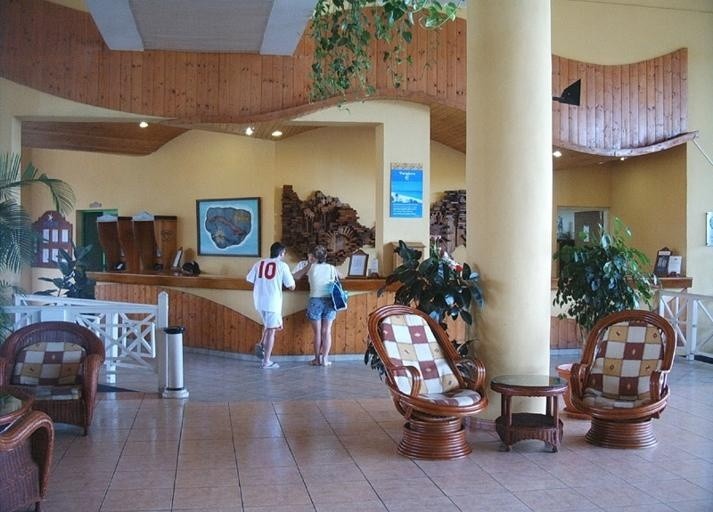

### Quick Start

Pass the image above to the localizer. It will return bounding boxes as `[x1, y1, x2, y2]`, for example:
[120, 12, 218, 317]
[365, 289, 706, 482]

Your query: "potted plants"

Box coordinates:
[551, 214, 658, 415]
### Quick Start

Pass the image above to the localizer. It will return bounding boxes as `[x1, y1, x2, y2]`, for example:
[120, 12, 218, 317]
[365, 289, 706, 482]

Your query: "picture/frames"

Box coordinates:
[195, 198, 262, 258]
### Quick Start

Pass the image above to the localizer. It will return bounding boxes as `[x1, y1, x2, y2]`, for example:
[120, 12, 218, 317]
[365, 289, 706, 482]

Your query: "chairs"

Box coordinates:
[0, 390, 55, 512]
[556, 309, 677, 449]
[368, 304, 489, 460]
[0, 320, 105, 435]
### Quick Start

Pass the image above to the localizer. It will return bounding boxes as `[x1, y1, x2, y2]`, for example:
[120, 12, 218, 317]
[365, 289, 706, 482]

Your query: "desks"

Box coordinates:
[491, 374, 567, 451]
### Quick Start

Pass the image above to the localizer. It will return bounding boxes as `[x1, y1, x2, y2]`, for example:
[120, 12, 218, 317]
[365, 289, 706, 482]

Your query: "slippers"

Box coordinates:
[255, 342, 264, 360]
[262, 361, 281, 370]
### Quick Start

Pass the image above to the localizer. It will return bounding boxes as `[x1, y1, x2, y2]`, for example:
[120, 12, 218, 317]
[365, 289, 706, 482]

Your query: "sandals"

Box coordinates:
[309, 360, 331, 368]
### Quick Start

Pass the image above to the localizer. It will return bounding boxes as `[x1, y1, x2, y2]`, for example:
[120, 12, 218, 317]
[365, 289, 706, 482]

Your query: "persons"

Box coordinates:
[435, 239, 462, 272]
[292, 250, 317, 281]
[305, 245, 338, 367]
[245, 242, 297, 369]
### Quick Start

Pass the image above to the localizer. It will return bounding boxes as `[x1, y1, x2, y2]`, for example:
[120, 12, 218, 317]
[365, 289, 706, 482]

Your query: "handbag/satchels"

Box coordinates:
[329, 279, 349, 313]
